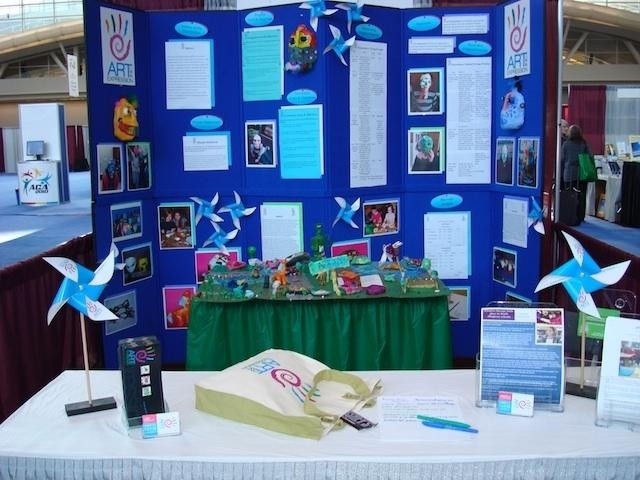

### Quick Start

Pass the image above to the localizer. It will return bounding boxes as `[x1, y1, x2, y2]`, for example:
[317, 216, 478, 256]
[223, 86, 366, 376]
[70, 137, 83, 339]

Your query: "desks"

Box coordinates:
[0, 370, 640, 480]
[190, 263, 451, 371]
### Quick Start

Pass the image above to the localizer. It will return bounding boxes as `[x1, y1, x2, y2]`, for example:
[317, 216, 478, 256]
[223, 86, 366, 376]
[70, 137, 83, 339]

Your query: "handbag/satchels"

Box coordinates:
[195, 348, 382, 440]
[578, 143, 598, 182]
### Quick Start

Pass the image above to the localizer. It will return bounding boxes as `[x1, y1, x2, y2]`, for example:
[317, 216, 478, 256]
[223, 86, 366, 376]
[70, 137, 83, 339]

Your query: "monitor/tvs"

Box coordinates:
[27, 141, 44, 160]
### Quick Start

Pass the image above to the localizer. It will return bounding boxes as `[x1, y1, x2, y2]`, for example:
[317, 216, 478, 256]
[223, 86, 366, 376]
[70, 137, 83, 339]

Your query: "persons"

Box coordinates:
[383, 205, 395, 226]
[536, 310, 561, 344]
[248, 134, 272, 163]
[559, 119, 570, 144]
[101, 153, 148, 236]
[161, 207, 177, 236]
[368, 208, 383, 225]
[411, 136, 438, 170]
[497, 144, 512, 184]
[178, 291, 190, 324]
[560, 125, 591, 221]
[172, 211, 188, 229]
[410, 73, 439, 112]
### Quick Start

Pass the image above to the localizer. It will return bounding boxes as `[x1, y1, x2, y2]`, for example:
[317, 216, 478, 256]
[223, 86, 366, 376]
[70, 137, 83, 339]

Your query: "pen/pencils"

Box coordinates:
[417, 415, 479, 433]
